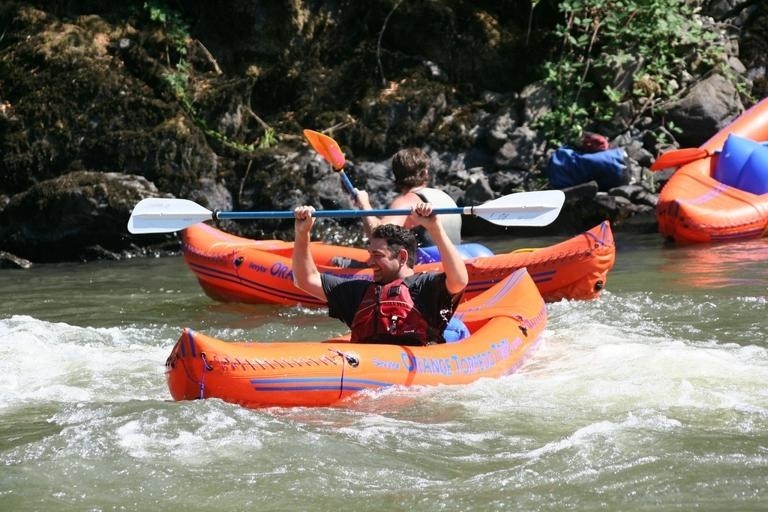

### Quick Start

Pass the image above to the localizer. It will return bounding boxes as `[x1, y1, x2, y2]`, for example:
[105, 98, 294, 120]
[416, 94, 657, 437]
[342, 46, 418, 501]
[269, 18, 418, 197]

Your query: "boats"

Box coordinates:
[655, 97, 768, 249]
[181, 221, 615, 310]
[165, 266, 547, 410]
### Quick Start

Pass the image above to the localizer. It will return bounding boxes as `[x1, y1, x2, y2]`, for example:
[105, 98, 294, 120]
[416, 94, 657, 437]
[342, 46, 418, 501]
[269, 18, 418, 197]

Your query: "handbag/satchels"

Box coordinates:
[547, 145, 633, 189]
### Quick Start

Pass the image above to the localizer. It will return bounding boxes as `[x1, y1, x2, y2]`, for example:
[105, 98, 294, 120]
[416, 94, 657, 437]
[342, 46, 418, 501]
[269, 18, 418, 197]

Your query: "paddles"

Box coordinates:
[126, 189, 565, 234]
[302, 128, 358, 202]
[650, 141, 768, 171]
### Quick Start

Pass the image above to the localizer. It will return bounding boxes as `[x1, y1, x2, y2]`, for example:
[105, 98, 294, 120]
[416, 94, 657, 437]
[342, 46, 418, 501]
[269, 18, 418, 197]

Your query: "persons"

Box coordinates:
[351, 148, 463, 245]
[292, 203, 470, 346]
